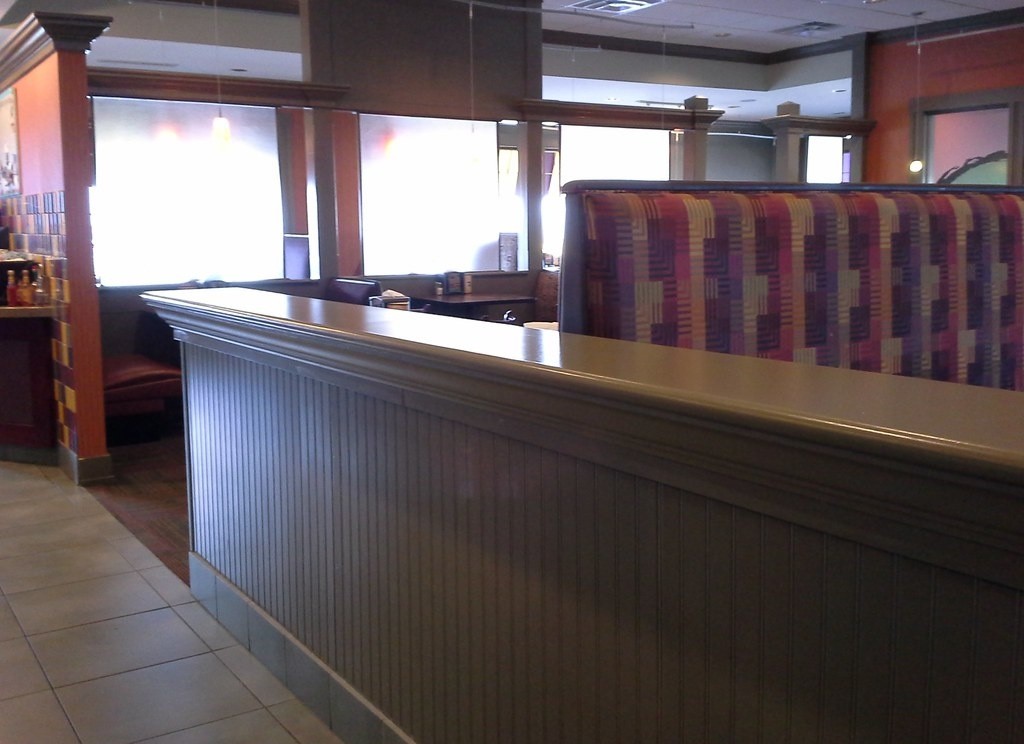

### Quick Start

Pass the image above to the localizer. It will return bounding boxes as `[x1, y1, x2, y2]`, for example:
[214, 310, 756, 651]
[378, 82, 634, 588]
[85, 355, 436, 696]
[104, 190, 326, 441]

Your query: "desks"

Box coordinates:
[409, 294, 538, 317]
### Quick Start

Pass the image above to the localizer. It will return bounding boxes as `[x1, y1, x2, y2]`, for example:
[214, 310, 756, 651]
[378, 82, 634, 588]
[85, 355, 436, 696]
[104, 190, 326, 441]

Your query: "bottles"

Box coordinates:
[436, 282, 444, 296]
[6, 264, 50, 306]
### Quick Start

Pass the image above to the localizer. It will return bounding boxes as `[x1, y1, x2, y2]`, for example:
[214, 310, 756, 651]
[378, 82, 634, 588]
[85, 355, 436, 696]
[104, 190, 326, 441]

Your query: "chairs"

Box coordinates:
[323, 275, 382, 305]
[532, 272, 560, 322]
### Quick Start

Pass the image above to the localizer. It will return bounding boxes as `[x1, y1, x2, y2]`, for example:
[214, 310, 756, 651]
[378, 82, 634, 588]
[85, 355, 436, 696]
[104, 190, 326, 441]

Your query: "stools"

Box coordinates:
[104, 346, 182, 416]
